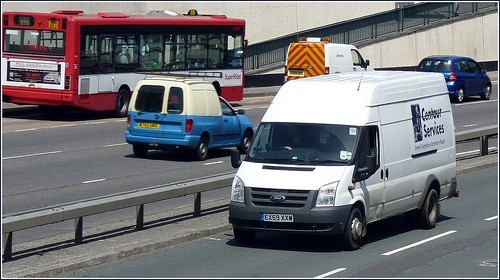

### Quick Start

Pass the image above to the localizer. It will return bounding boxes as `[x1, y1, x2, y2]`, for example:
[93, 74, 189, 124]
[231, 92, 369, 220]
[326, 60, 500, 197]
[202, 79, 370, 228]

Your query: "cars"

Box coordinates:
[415, 55, 492, 103]
[126, 75, 256, 160]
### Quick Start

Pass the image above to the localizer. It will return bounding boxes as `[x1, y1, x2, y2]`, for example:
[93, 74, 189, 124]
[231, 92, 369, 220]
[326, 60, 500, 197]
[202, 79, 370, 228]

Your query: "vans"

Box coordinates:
[228, 70, 459, 250]
[284, 36, 374, 83]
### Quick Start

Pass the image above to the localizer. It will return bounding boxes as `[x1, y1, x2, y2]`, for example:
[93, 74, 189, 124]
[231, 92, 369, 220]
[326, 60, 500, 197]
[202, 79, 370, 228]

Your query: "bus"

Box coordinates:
[1, 9, 249, 117]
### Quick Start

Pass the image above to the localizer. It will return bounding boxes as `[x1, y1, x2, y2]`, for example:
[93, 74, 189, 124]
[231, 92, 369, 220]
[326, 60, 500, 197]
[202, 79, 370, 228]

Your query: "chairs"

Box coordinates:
[9, 38, 224, 74]
